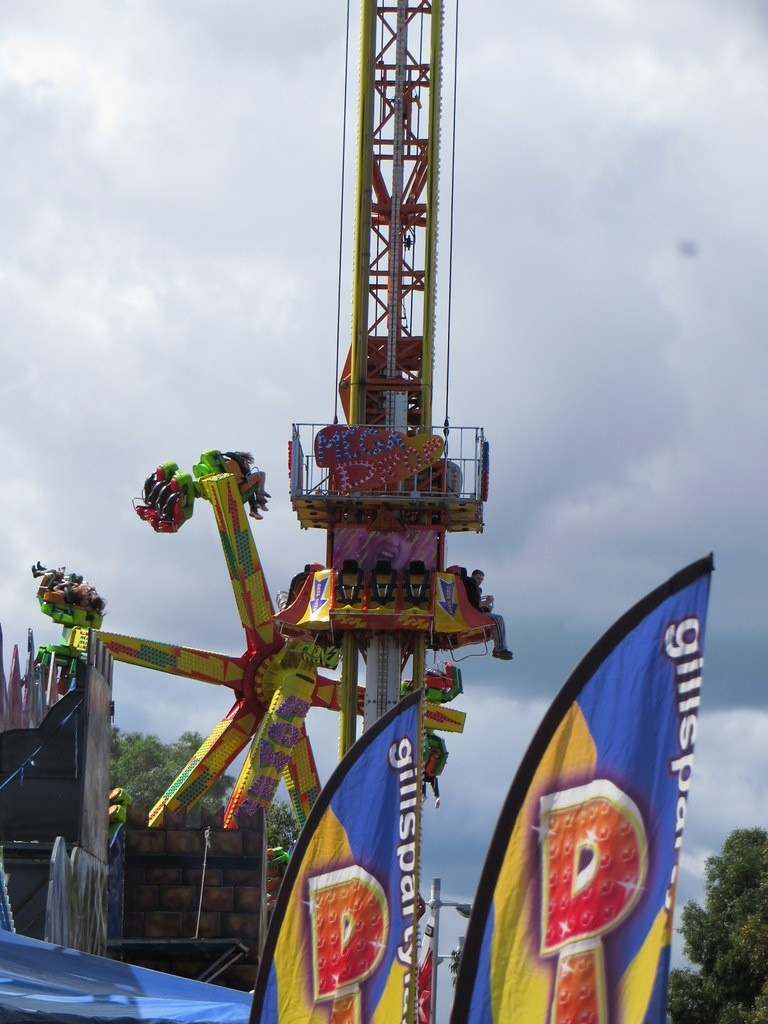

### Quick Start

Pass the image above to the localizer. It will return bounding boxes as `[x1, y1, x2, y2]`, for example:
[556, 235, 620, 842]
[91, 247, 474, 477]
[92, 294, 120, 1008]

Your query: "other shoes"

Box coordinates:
[31, 565, 42, 579]
[254, 490, 272, 503]
[36, 561, 45, 571]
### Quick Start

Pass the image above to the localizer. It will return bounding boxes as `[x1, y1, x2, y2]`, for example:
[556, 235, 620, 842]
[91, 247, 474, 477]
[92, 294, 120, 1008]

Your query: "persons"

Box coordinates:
[468, 570, 513, 660]
[32, 561, 105, 611]
[235, 452, 271, 519]
[423, 727, 447, 805]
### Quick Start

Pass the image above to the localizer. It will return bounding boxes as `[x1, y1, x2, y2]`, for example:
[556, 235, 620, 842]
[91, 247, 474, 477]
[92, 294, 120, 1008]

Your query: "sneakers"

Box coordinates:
[254, 499, 270, 512]
[248, 510, 264, 520]
[434, 797, 441, 809]
[492, 649, 514, 660]
